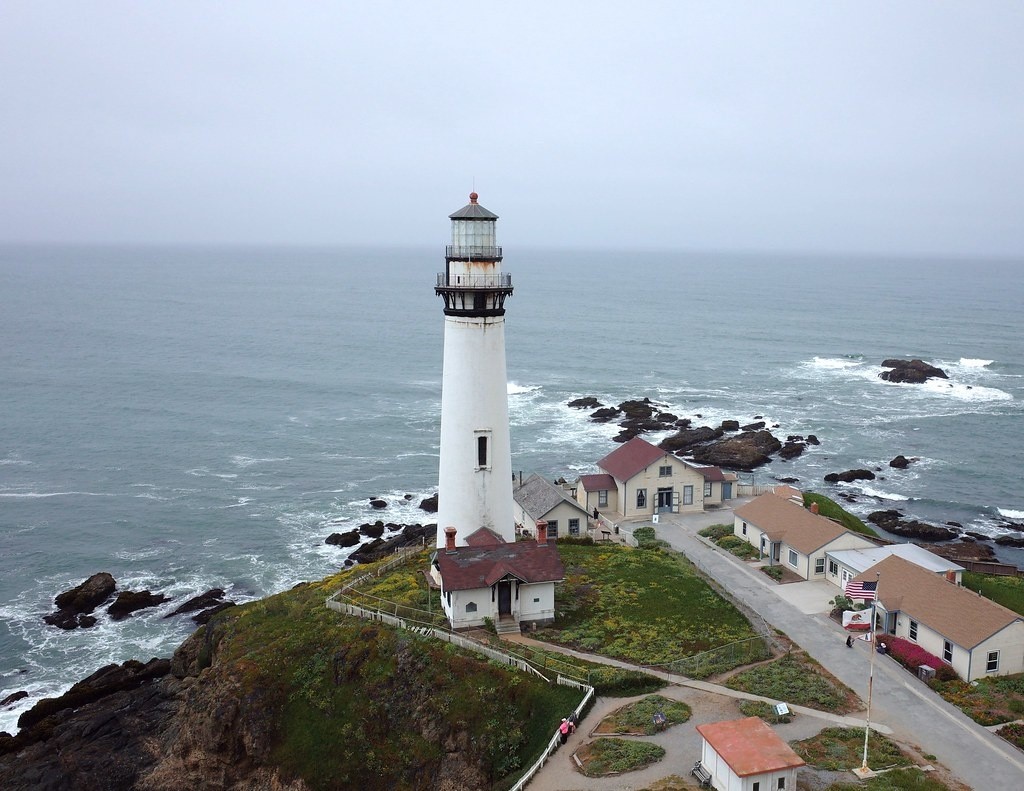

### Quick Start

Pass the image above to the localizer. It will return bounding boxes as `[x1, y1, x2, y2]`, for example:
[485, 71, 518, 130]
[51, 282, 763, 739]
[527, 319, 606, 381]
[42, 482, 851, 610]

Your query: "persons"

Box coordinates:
[846, 636, 855, 648]
[560, 718, 568, 744]
[593, 507, 599, 524]
[569, 710, 577, 734]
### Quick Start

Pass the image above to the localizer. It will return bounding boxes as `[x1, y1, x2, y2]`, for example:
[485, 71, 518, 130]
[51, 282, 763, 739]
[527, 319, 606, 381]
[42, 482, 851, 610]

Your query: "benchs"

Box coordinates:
[691, 763, 712, 789]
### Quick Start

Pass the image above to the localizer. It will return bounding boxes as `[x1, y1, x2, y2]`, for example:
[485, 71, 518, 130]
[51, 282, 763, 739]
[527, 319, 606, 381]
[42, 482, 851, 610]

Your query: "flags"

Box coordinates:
[845, 581, 878, 600]
[842, 608, 872, 629]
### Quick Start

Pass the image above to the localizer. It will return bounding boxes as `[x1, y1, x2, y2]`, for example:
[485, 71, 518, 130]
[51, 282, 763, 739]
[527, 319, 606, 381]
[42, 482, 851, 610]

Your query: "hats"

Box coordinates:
[562, 718, 566, 721]
